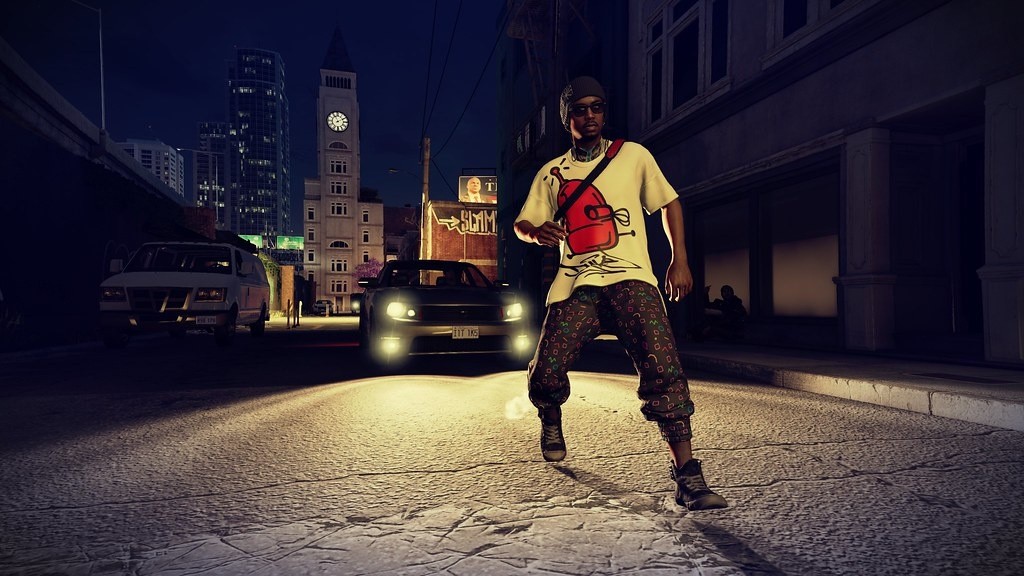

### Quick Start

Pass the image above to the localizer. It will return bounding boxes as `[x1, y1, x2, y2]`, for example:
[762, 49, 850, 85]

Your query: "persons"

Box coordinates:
[460, 176, 490, 202]
[439, 264, 467, 287]
[714, 284, 747, 317]
[512, 76, 729, 511]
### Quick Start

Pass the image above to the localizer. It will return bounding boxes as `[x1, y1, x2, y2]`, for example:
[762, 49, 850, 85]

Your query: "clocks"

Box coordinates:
[326, 111, 349, 133]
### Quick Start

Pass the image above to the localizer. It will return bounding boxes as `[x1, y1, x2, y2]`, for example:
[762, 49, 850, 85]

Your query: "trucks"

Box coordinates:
[350, 293, 364, 314]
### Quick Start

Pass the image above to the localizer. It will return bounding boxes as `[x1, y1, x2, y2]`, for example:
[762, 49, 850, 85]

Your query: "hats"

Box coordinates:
[560, 76, 606, 133]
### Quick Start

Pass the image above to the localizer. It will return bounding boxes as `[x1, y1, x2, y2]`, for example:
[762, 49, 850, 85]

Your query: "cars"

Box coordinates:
[312, 300, 334, 315]
[356, 260, 532, 369]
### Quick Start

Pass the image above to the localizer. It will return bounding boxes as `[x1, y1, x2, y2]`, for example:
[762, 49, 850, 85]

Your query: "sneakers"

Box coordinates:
[538, 405, 567, 462]
[668, 458, 728, 510]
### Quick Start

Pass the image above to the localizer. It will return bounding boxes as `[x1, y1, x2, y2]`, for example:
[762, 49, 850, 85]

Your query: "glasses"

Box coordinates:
[569, 101, 606, 116]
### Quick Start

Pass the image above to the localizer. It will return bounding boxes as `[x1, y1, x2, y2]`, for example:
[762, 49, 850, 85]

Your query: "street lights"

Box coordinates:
[389, 168, 424, 259]
[177, 148, 224, 221]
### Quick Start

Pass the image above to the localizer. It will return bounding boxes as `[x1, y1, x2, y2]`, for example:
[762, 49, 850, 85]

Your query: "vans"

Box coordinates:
[98, 242, 271, 347]
